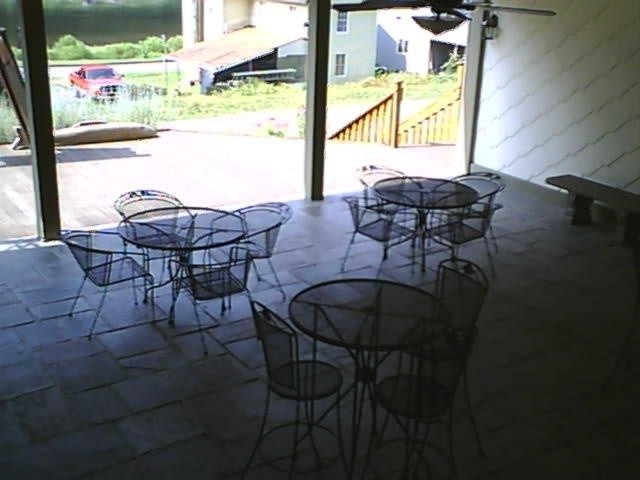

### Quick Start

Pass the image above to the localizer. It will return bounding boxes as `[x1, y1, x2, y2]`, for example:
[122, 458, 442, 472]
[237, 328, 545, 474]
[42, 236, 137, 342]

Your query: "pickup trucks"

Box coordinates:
[70, 63, 127, 103]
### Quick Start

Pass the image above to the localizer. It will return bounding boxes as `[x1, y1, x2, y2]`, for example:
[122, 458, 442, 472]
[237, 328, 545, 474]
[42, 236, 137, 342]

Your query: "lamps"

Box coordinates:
[480, 13, 499, 41]
[409, 14, 467, 34]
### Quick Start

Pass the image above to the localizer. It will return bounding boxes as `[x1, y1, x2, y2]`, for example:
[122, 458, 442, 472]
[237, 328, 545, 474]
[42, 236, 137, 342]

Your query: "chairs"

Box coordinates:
[209, 201, 294, 310]
[420, 201, 504, 284]
[428, 170, 506, 258]
[358, 164, 425, 247]
[61, 227, 157, 340]
[240, 299, 349, 479]
[359, 323, 481, 478]
[341, 195, 417, 282]
[389, 257, 491, 453]
[166, 240, 272, 357]
[113, 189, 197, 303]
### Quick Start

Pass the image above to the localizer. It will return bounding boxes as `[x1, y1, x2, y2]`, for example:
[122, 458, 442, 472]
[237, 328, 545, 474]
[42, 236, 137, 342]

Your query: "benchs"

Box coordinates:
[545, 173, 640, 244]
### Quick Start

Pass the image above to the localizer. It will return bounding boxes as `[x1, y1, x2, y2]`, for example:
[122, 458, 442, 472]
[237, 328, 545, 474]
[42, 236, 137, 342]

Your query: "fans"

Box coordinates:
[331, 0, 556, 22]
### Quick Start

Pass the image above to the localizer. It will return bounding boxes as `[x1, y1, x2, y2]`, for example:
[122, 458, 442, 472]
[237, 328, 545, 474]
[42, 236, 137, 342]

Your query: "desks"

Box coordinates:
[116, 205, 248, 326]
[370, 177, 481, 273]
[287, 277, 452, 478]
